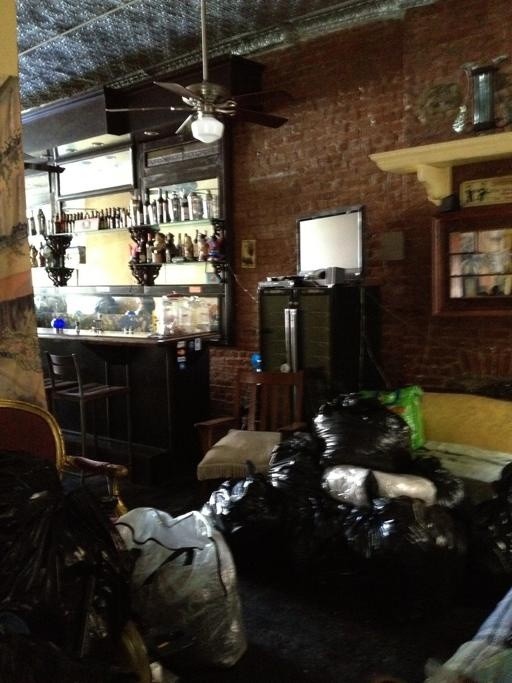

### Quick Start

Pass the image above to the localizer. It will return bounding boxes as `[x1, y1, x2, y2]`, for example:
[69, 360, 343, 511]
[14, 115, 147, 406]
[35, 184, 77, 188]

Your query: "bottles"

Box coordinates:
[25, 184, 220, 266]
[155, 294, 213, 337]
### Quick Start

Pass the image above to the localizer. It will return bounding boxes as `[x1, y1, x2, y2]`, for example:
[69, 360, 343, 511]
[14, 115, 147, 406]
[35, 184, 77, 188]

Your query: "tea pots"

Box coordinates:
[464, 54, 505, 132]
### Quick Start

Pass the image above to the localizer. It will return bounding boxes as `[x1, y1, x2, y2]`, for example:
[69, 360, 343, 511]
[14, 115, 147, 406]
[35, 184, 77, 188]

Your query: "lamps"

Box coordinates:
[188, 105, 226, 143]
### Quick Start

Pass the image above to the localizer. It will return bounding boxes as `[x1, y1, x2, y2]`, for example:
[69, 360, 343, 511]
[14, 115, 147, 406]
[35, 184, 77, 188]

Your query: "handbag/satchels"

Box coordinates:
[114, 506, 248, 669]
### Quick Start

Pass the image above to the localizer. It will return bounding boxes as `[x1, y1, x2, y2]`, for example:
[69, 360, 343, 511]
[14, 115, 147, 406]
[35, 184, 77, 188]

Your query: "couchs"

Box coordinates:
[412, 386, 512, 491]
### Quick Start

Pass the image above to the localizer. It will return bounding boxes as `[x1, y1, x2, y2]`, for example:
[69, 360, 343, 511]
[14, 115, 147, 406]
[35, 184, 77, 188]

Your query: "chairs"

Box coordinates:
[192, 367, 306, 483]
[0, 396, 132, 526]
[45, 349, 135, 471]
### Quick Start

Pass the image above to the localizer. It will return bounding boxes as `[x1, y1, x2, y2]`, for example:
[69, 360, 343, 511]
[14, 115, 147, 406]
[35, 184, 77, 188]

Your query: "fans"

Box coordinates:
[104, 0, 294, 137]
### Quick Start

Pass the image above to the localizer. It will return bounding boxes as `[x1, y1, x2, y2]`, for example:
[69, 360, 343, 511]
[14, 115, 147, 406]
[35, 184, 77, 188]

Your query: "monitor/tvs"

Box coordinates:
[296, 204, 365, 277]
